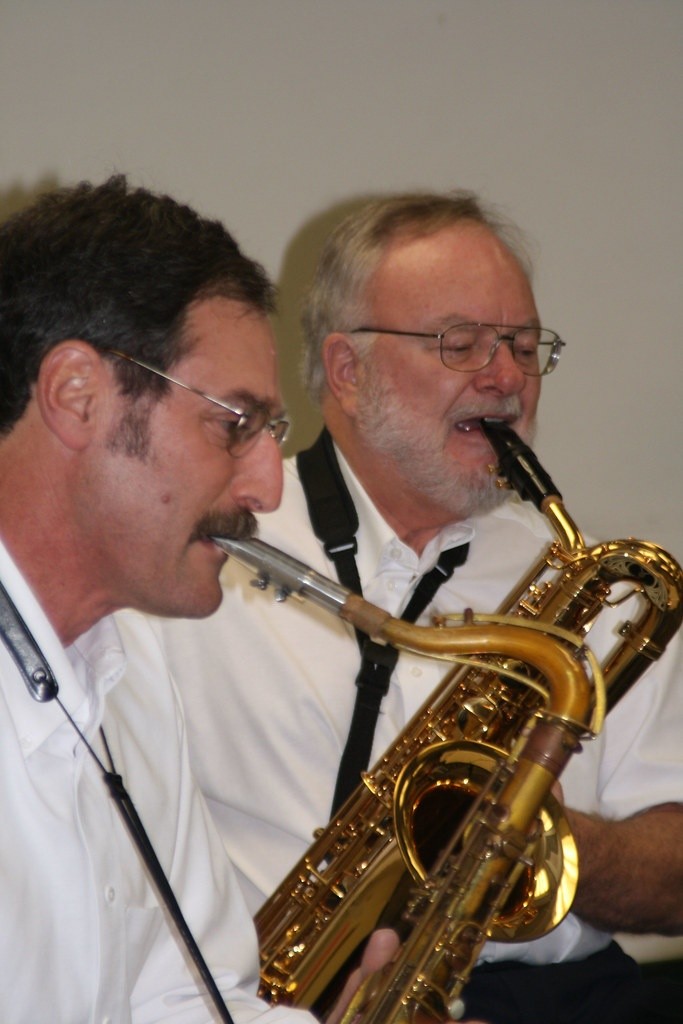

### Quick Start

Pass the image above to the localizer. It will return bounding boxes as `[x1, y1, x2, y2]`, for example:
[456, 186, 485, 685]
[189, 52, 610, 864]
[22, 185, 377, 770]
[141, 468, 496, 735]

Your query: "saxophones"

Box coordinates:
[252, 419, 683, 1023]
[211, 538, 605, 1024]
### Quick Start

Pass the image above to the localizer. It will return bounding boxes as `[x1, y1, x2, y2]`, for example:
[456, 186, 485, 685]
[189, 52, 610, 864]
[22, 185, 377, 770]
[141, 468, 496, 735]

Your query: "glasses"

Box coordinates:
[349, 320, 566, 379]
[101, 347, 290, 460]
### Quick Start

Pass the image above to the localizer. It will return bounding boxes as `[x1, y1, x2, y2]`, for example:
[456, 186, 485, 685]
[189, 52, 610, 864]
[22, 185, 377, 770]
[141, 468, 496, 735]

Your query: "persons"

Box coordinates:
[158, 189, 683, 1024]
[1, 173, 446, 1024]
